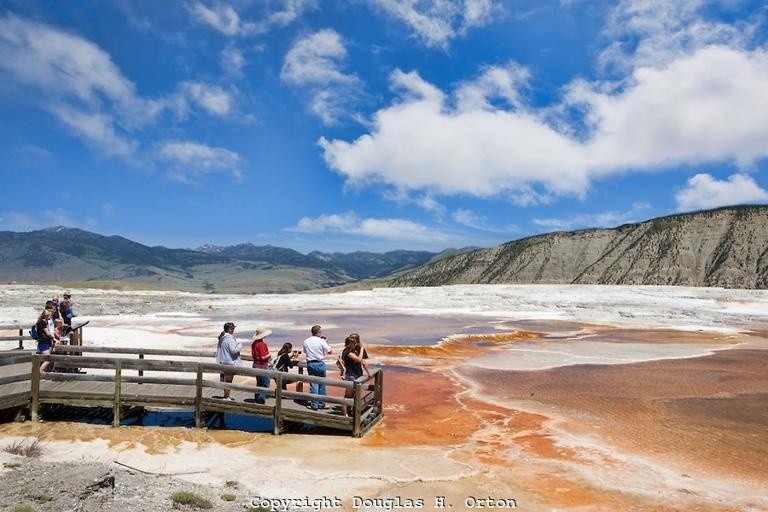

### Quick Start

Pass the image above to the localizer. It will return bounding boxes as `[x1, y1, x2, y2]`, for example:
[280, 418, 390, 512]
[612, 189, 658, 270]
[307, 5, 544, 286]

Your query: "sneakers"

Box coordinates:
[319, 404, 329, 409]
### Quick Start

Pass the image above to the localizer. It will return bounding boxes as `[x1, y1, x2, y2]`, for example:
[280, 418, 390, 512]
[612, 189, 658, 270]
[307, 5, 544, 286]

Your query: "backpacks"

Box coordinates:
[29, 325, 39, 340]
[269, 353, 284, 370]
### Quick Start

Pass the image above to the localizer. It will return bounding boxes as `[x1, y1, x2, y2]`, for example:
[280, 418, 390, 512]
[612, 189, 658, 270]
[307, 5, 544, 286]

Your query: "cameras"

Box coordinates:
[320, 336, 326, 340]
[294, 351, 301, 354]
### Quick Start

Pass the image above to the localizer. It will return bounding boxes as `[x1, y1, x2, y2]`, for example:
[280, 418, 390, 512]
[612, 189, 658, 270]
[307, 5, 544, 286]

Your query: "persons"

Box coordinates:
[36, 291, 81, 373]
[273, 343, 298, 389]
[348, 333, 372, 378]
[302, 325, 332, 411]
[341, 337, 373, 417]
[251, 327, 273, 404]
[215, 322, 242, 401]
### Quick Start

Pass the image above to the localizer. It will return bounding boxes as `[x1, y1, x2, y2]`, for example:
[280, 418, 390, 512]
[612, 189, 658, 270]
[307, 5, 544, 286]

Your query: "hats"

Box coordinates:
[223, 323, 237, 329]
[252, 327, 272, 340]
